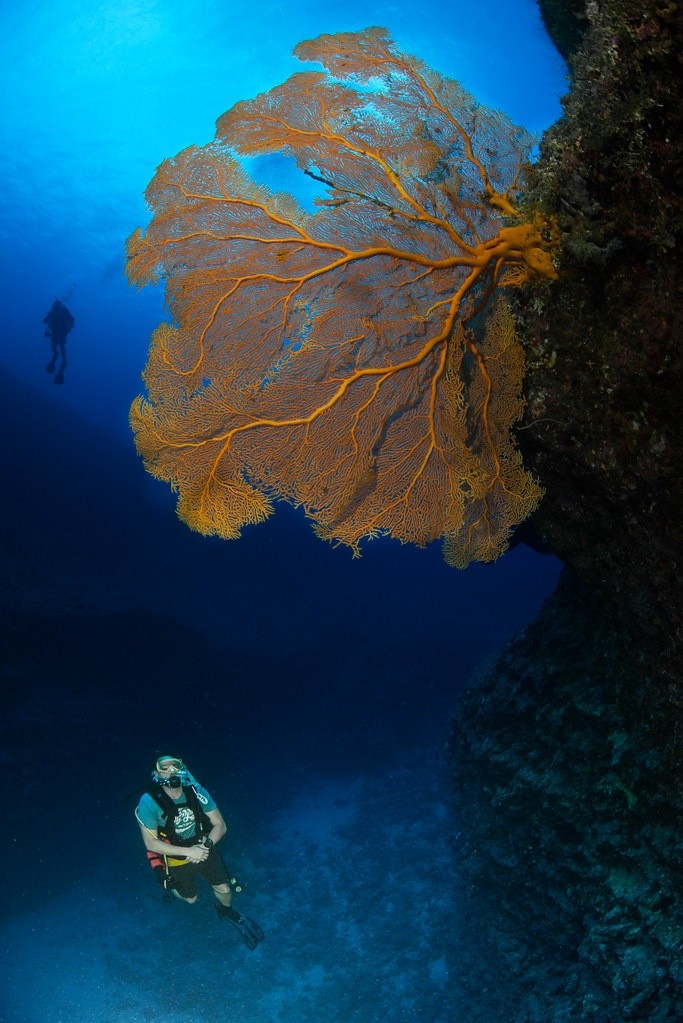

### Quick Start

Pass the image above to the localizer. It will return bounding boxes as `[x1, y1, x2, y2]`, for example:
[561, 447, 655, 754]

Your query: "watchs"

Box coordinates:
[203, 838, 214, 849]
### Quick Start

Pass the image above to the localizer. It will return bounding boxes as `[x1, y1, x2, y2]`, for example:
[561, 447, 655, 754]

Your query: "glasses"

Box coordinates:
[156, 759, 183, 773]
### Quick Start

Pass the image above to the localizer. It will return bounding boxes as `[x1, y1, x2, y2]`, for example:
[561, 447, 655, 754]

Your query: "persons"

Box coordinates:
[135, 756, 265, 951]
[44, 300, 75, 385]
